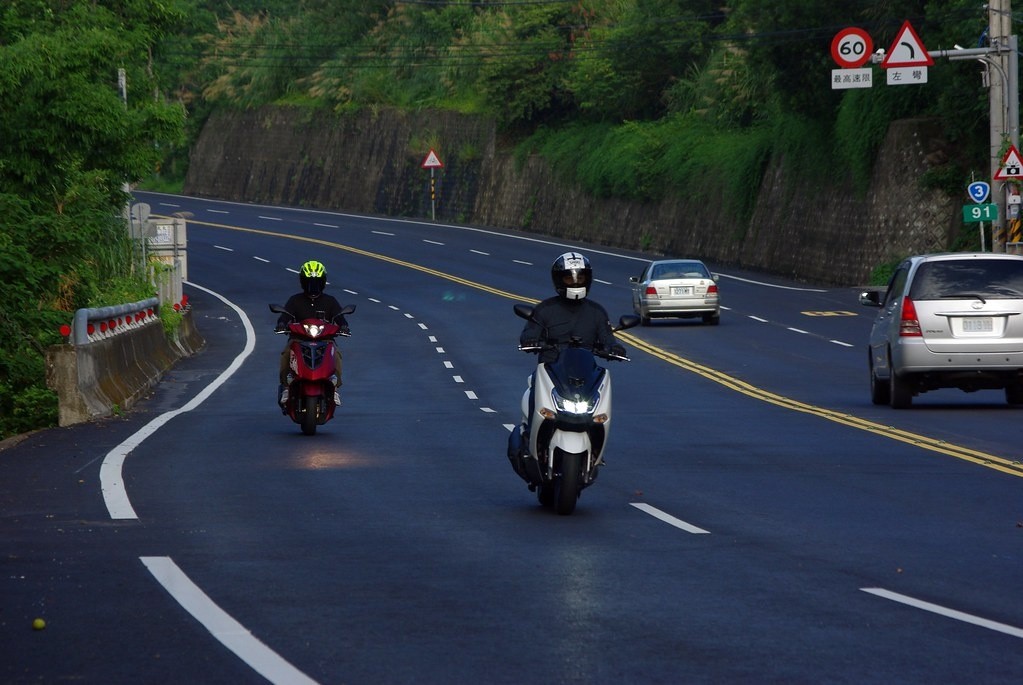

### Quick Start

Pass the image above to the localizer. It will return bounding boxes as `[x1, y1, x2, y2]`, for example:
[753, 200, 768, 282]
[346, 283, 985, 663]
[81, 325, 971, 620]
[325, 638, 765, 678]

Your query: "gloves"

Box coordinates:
[608, 344, 627, 362]
[340, 326, 351, 338]
[275, 323, 289, 336]
[520, 339, 539, 356]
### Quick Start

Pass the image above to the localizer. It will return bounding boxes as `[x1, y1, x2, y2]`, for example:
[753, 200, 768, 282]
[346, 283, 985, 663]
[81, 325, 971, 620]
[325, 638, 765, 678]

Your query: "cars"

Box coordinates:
[628, 259, 722, 325]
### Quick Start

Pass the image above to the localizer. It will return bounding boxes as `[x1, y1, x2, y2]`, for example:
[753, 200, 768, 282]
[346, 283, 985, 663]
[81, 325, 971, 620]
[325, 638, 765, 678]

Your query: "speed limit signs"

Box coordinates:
[831, 27, 874, 69]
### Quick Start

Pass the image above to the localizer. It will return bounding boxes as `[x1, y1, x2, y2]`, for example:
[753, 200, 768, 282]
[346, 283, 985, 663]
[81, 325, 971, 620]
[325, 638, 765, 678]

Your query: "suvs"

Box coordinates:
[857, 252, 1023, 408]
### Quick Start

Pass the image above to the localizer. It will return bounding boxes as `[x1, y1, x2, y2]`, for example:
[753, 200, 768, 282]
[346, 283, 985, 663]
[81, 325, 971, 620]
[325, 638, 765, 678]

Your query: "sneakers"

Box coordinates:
[280, 389, 290, 403]
[334, 391, 341, 406]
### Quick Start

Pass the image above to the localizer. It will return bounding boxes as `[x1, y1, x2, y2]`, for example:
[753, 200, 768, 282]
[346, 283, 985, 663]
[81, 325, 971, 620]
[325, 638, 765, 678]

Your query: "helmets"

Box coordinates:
[550, 252, 593, 301]
[299, 260, 327, 294]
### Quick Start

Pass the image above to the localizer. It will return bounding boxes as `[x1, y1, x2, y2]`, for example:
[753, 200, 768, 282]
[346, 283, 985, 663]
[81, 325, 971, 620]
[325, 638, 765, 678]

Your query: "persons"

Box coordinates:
[276, 261, 351, 405]
[520, 252, 627, 467]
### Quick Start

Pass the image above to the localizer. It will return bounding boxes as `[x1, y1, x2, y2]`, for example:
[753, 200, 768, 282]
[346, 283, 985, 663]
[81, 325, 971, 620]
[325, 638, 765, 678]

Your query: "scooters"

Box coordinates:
[268, 303, 357, 436]
[506, 302, 642, 517]
